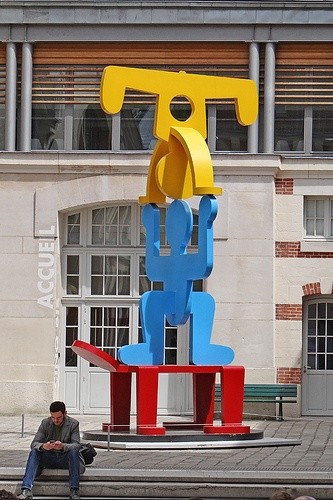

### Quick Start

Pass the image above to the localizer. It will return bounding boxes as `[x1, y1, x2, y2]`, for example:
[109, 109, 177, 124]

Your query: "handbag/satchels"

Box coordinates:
[77, 442, 98, 474]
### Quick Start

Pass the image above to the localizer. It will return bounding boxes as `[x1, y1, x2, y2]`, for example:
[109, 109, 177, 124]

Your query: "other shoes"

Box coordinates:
[70, 488, 80, 500]
[16, 488, 33, 500]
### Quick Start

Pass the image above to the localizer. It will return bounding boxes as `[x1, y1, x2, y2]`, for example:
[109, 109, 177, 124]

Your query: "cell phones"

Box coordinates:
[50, 440, 55, 444]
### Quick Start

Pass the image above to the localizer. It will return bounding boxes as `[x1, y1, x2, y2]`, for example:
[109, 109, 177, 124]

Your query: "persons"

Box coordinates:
[18, 401, 88, 500]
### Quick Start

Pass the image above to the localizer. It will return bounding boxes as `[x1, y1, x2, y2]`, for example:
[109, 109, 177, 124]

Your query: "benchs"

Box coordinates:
[214, 384, 297, 420]
